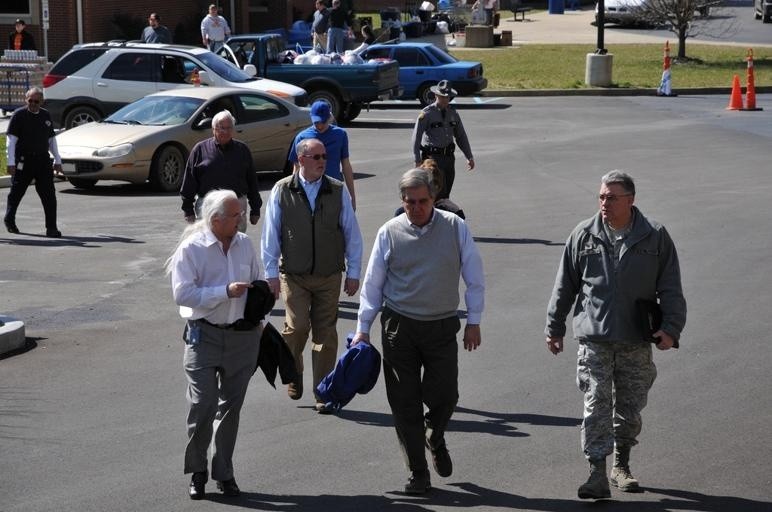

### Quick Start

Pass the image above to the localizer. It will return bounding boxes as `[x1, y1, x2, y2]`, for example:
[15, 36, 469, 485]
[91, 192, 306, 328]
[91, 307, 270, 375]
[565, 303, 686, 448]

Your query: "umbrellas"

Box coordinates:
[218, 479, 240, 496]
[189, 479, 204, 499]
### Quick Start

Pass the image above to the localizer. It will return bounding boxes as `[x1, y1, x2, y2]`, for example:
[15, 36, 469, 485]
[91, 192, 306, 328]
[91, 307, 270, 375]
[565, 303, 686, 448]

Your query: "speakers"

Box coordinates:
[578, 460, 610, 498]
[610, 454, 638, 491]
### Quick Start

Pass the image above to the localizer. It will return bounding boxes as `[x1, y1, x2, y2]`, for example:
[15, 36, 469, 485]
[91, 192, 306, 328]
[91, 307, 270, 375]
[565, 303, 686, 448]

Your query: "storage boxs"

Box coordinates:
[510, 7, 533, 21]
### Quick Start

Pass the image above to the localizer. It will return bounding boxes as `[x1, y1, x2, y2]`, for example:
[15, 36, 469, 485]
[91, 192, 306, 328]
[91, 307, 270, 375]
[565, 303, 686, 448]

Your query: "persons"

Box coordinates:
[4, 87, 61, 239]
[543, 168, 687, 500]
[410, 80, 475, 202]
[260, 140, 364, 414]
[393, 159, 465, 221]
[311, 1, 497, 56]
[201, 3, 231, 53]
[350, 167, 485, 494]
[140, 12, 172, 64]
[8, 19, 35, 49]
[287, 97, 356, 213]
[179, 109, 263, 234]
[164, 188, 266, 497]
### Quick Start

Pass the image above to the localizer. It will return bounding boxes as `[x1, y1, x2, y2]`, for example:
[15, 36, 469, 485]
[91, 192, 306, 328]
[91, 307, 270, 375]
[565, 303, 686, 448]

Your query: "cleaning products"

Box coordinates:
[3, 69, 37, 112]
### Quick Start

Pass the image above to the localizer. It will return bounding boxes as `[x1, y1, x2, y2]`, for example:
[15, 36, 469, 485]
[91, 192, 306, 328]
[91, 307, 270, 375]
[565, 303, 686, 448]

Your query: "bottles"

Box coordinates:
[311, 101, 328, 122]
[431, 80, 457, 97]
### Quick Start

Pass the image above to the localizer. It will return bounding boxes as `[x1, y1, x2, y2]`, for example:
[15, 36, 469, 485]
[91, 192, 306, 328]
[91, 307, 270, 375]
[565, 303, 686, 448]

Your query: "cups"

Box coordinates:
[303, 154, 328, 160]
[600, 194, 628, 200]
[27, 98, 39, 104]
[224, 210, 244, 217]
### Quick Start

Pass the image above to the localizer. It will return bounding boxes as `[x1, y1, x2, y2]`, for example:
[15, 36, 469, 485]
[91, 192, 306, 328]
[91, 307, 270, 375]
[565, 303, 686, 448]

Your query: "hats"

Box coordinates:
[593, 0, 709, 24]
[353, 37, 487, 107]
[38, 84, 323, 189]
[747, 3, 772, 24]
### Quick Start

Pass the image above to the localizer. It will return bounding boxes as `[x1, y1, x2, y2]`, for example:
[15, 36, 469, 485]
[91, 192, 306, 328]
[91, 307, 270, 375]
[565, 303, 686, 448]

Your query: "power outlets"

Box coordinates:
[211, 32, 398, 119]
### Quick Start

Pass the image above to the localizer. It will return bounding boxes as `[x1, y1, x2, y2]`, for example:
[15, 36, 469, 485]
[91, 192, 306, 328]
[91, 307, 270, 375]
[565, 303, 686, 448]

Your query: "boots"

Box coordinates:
[45, 39, 301, 128]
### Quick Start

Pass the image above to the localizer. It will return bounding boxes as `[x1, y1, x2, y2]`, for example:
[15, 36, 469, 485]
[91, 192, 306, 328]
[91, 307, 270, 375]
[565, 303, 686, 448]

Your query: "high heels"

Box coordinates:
[724, 76, 743, 112]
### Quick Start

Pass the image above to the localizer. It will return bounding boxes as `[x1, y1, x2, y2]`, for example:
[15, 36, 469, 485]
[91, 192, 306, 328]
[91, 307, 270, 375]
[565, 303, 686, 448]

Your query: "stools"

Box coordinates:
[432, 147, 450, 154]
[199, 318, 237, 329]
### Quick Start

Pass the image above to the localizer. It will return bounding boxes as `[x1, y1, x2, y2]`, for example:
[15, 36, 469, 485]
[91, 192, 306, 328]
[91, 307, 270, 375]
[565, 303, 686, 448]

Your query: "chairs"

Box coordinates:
[426, 437, 452, 476]
[46, 229, 61, 237]
[4, 218, 19, 232]
[405, 471, 430, 492]
[288, 374, 302, 399]
[316, 401, 325, 412]
